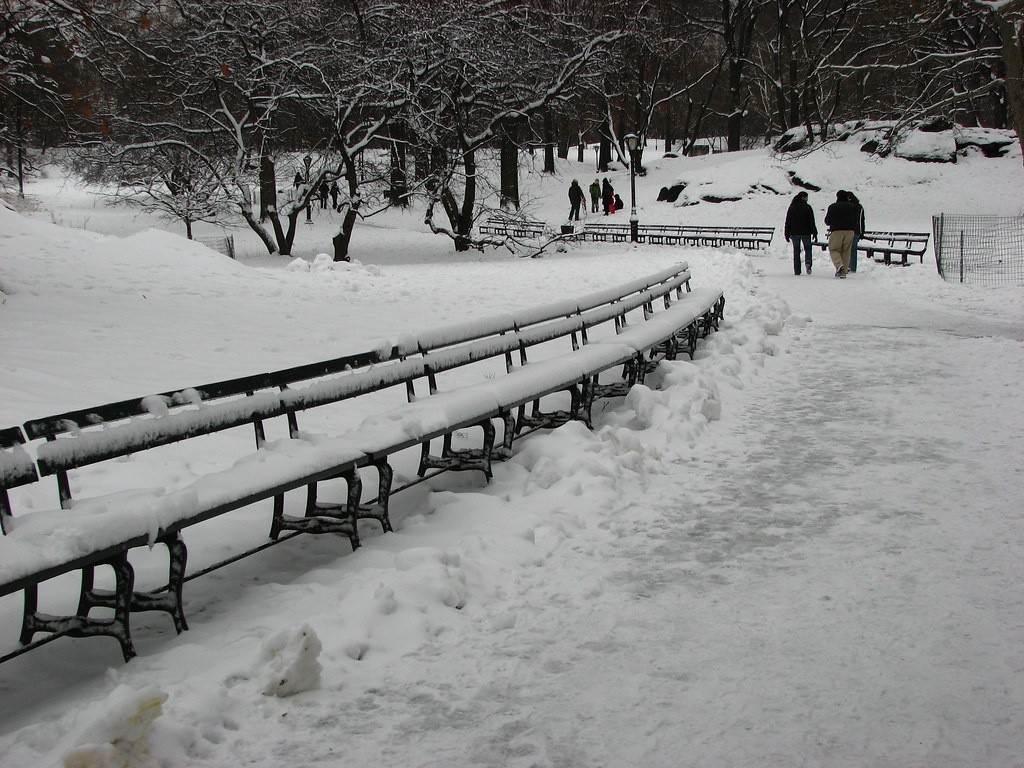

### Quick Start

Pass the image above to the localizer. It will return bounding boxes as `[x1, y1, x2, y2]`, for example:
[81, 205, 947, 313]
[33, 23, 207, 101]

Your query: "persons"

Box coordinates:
[330, 181, 342, 209]
[784, 191, 818, 275]
[846, 191, 865, 273]
[293, 172, 304, 190]
[589, 179, 601, 213]
[318, 181, 329, 209]
[600, 178, 615, 216]
[568, 179, 586, 221]
[602, 194, 623, 213]
[825, 190, 857, 278]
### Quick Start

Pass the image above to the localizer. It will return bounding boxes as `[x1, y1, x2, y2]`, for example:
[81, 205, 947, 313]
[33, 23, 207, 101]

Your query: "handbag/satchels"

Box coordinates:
[609, 202, 616, 213]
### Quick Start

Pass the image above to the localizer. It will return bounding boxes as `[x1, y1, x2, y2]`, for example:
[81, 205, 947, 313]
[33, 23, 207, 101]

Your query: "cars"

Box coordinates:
[918, 116, 954, 132]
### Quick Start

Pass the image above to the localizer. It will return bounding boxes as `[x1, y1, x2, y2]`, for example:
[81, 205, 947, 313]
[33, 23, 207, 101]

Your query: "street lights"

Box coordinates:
[304, 155, 314, 225]
[624, 134, 642, 245]
[593, 144, 599, 169]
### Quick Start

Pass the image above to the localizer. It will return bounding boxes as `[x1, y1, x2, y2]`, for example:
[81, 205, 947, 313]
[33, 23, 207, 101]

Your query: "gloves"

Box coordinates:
[812, 234, 818, 243]
[786, 235, 790, 242]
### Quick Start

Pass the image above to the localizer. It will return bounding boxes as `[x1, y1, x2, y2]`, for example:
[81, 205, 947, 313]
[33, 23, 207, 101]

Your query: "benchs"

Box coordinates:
[0, 217, 931, 665]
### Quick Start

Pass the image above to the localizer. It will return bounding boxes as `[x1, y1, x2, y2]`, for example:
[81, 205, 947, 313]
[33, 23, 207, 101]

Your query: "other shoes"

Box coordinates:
[833, 266, 845, 277]
[847, 267, 855, 273]
[805, 263, 811, 275]
[840, 277, 846, 278]
